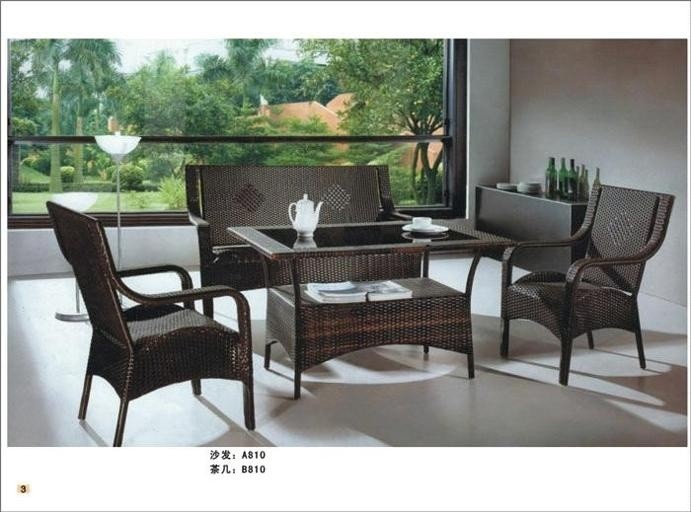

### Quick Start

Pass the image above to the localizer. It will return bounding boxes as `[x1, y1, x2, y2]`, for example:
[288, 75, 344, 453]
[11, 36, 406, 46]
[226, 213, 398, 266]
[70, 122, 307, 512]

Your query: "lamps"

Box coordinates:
[50, 189, 99, 321]
[93, 129, 143, 271]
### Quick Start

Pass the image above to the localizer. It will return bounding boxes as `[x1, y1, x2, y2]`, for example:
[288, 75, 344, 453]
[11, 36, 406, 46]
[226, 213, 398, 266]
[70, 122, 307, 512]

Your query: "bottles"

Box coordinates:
[544, 156, 578, 202]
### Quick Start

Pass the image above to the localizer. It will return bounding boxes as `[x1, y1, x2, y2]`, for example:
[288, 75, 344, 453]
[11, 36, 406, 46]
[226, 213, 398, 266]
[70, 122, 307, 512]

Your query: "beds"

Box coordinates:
[224, 218, 518, 400]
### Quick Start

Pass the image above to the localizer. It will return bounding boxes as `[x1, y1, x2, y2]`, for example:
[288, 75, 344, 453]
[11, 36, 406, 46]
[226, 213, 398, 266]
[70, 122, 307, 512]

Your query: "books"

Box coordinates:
[306, 279, 418, 303]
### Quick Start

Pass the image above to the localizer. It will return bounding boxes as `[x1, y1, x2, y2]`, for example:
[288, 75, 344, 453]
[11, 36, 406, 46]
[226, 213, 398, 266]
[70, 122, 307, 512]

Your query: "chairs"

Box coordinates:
[45, 199, 255, 447]
[500, 183, 676, 387]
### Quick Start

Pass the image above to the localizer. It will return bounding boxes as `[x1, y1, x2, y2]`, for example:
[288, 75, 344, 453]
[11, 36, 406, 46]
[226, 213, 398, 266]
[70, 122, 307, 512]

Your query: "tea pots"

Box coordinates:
[288, 192, 323, 237]
[292, 237, 316, 249]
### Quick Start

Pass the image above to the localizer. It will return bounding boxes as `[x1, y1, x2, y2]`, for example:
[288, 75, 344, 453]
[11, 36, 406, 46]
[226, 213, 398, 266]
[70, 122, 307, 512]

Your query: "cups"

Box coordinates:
[411, 217, 432, 229]
[411, 239, 431, 243]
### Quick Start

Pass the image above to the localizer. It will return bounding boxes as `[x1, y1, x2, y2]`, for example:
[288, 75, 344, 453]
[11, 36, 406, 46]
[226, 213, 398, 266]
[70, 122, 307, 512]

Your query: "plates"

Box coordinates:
[400, 232, 450, 240]
[495, 181, 540, 195]
[401, 223, 450, 234]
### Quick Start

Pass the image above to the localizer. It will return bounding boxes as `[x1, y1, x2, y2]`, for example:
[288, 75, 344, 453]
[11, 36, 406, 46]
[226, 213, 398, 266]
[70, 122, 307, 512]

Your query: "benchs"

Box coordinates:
[184, 164, 419, 330]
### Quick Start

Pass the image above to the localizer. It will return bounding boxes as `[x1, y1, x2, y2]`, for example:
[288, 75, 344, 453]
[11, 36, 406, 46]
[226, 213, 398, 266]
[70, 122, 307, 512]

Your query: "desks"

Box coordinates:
[474, 184, 589, 274]
